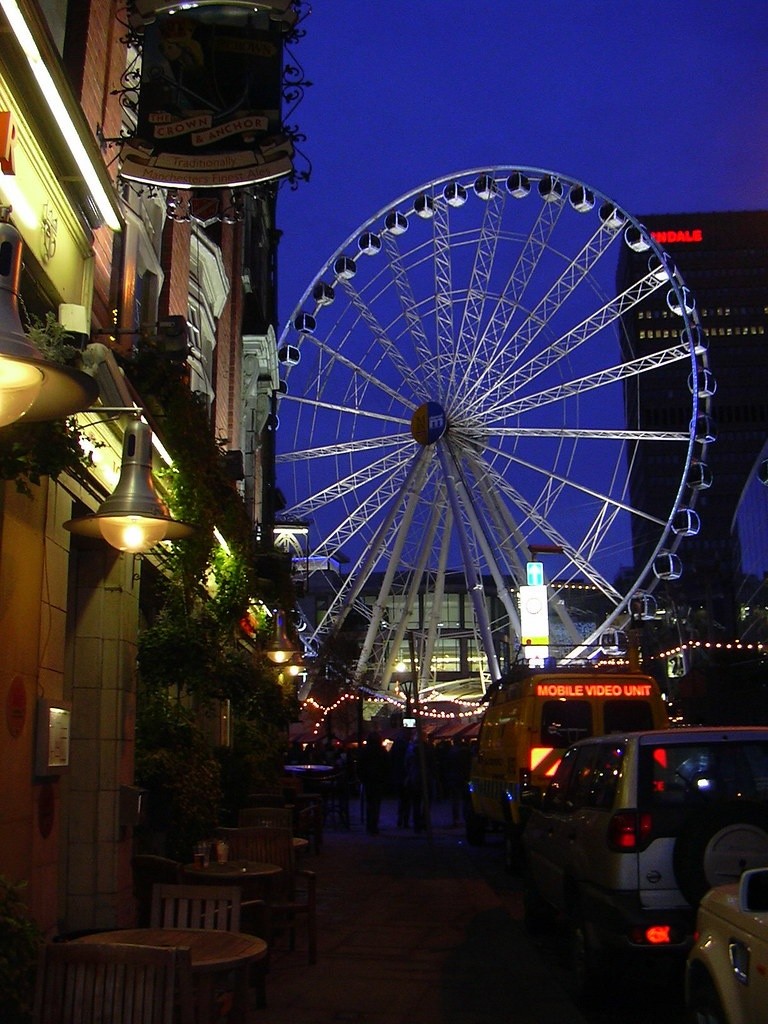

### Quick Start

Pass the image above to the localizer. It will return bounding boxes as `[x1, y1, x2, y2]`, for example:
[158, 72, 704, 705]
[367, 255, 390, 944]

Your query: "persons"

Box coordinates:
[305, 732, 476, 833]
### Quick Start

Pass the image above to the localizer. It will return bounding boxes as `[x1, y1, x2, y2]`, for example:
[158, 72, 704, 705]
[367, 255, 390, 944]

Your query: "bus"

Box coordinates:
[464, 655, 671, 878]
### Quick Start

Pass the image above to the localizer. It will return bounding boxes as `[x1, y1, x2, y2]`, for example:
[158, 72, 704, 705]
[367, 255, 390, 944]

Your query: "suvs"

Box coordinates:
[514, 722, 767, 986]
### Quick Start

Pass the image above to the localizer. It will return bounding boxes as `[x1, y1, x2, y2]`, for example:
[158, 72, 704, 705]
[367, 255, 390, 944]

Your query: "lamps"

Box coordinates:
[0, 216, 102, 431]
[274, 641, 312, 677]
[63, 396, 205, 551]
[234, 601, 297, 663]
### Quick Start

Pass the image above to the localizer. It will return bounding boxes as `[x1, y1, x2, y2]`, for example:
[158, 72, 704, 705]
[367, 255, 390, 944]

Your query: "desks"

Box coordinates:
[214, 827, 290, 852]
[179, 859, 280, 935]
[235, 835, 309, 866]
[67, 928, 269, 1024]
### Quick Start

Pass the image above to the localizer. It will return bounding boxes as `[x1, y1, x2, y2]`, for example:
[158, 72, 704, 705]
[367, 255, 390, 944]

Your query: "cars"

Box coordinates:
[682, 867, 768, 1024]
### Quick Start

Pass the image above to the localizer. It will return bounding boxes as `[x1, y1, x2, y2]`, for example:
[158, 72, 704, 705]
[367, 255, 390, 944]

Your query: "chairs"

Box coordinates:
[29, 779, 330, 1023]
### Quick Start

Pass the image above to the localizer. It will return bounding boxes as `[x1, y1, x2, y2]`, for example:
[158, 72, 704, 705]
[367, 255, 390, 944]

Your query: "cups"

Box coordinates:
[215, 840, 229, 865]
[198, 841, 212, 868]
[194, 854, 205, 868]
